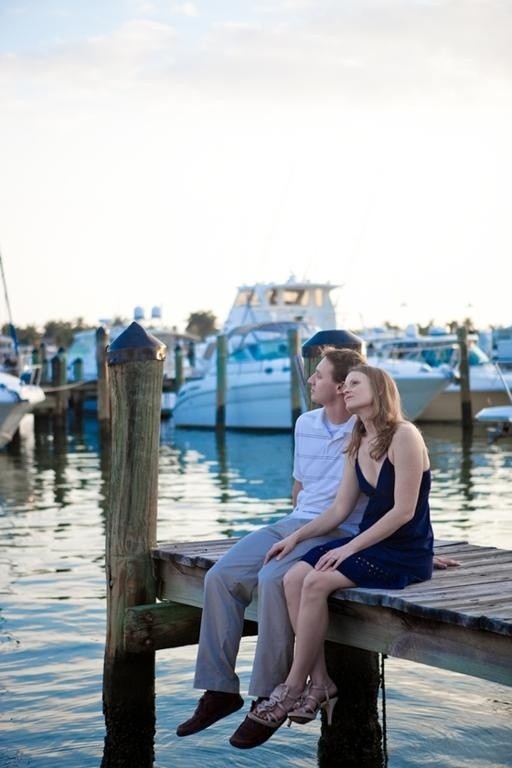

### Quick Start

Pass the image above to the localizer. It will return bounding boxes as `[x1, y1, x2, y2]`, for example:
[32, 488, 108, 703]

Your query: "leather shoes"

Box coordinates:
[228, 695, 288, 749]
[176, 690, 244, 737]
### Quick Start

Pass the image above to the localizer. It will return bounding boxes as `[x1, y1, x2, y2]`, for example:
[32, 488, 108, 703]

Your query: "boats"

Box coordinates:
[0, 363, 49, 455]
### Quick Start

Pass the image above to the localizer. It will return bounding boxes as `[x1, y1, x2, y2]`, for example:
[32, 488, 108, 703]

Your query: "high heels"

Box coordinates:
[247, 681, 310, 729]
[286, 676, 340, 727]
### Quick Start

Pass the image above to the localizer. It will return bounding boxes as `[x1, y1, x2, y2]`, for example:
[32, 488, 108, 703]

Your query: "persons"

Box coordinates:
[247, 364, 434, 728]
[176, 345, 368, 749]
